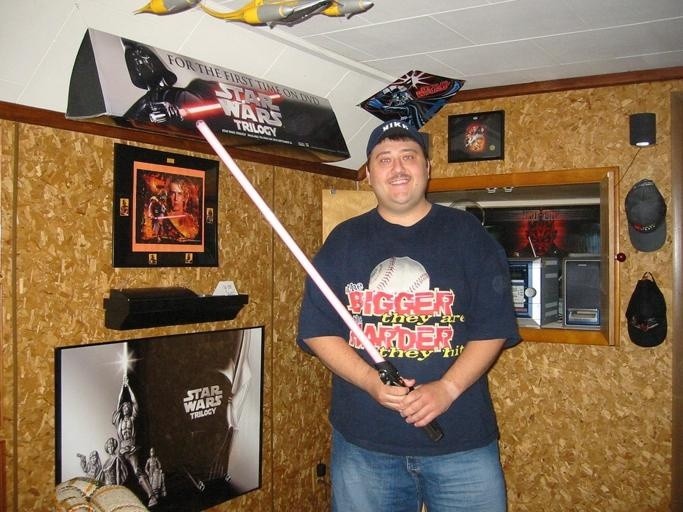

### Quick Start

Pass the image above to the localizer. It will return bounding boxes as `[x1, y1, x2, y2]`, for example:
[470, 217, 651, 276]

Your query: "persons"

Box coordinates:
[96, 438, 129, 486]
[207, 210, 213, 223]
[75, 450, 104, 482]
[144, 447, 167, 498]
[151, 179, 198, 242]
[295, 118, 524, 510]
[111, 376, 158, 509]
[119, 200, 128, 216]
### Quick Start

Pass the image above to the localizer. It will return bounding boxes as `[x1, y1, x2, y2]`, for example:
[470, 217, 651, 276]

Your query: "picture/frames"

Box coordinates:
[110, 143, 218, 268]
[54, 324, 266, 512]
[447, 109, 504, 163]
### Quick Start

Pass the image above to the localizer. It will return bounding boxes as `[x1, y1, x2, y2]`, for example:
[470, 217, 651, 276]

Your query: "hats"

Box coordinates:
[366, 118, 428, 156]
[624, 179, 667, 252]
[626, 271, 671, 348]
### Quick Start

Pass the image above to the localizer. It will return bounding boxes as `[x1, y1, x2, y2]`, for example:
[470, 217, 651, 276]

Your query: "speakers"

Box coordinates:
[629, 112, 657, 148]
